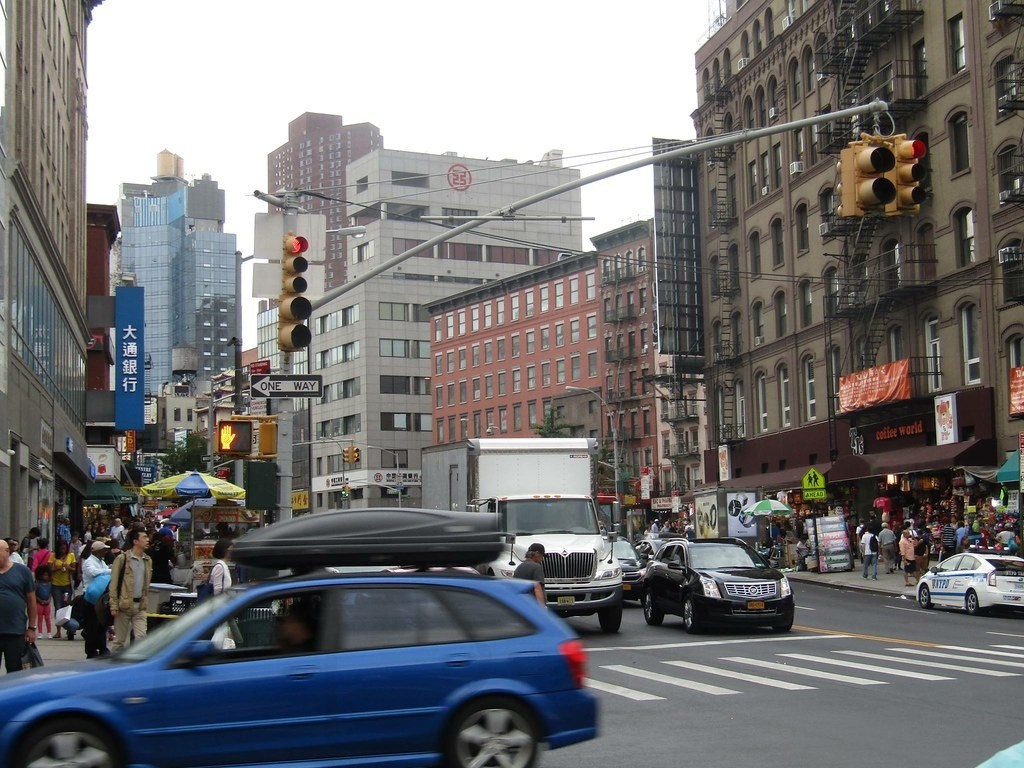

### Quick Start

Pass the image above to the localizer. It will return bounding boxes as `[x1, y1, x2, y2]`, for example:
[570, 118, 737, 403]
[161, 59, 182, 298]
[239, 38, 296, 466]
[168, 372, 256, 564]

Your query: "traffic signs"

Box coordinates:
[250, 373, 324, 399]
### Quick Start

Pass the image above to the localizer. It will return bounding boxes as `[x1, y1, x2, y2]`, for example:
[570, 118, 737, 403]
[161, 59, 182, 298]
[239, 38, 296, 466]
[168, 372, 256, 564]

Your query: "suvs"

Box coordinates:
[0, 505, 598, 768]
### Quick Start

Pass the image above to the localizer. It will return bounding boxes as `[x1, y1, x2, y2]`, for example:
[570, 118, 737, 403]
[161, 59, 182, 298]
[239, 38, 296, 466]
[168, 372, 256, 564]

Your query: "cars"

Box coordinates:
[603, 534, 669, 601]
[643, 535, 794, 634]
[914, 544, 1024, 616]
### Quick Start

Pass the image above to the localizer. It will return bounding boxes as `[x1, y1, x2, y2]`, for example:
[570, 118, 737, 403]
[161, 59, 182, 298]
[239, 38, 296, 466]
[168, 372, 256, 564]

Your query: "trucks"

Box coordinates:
[420, 438, 623, 634]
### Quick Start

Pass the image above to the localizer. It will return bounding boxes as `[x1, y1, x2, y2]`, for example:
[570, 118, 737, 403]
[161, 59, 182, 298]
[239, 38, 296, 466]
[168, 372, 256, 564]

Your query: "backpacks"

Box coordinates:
[961, 530, 970, 549]
[870, 535, 877, 552]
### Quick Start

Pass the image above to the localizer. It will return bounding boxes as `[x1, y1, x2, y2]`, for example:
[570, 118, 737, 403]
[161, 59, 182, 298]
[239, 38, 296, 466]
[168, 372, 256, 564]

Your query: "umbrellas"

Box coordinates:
[142, 470, 247, 571]
[744, 498, 793, 539]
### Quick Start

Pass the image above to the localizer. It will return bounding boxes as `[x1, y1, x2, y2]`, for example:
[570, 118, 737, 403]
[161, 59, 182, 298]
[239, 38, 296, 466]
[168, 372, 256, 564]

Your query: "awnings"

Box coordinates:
[997, 449, 1021, 483]
[82, 482, 137, 506]
[680, 461, 836, 503]
[828, 439, 997, 483]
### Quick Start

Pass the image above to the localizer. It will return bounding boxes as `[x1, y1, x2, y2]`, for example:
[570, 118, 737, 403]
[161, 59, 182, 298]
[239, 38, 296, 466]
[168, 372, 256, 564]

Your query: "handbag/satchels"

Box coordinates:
[105, 552, 115, 564]
[210, 621, 235, 650]
[1008, 533, 1020, 551]
[34, 564, 52, 581]
[940, 470, 987, 498]
[20, 641, 43, 670]
[84, 574, 115, 626]
[55, 605, 73, 626]
[196, 583, 214, 602]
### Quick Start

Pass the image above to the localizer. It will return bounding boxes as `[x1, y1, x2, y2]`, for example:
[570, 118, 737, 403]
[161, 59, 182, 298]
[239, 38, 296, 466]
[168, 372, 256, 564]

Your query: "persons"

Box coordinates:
[273, 606, 321, 654]
[514, 543, 548, 611]
[0, 516, 237, 674]
[644, 516, 696, 539]
[848, 511, 1021, 586]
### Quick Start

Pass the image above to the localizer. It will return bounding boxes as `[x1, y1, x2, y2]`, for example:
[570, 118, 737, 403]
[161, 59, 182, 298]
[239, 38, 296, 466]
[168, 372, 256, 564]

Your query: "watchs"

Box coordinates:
[28, 626, 37, 631]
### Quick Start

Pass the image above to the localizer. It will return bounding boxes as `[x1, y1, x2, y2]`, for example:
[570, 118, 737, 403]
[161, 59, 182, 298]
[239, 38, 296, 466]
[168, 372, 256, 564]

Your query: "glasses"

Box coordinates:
[8, 543, 16, 548]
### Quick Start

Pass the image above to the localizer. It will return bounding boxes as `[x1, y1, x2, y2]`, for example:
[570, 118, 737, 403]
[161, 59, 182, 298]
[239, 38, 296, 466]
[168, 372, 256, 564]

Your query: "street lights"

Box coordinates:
[565, 385, 621, 535]
[368, 445, 402, 508]
[461, 417, 489, 437]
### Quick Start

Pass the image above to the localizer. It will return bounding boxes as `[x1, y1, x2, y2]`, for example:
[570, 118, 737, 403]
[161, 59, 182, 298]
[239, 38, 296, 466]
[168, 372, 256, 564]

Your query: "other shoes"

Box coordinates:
[905, 583, 913, 586]
[37, 632, 75, 640]
[861, 576, 877, 581]
[81, 630, 87, 637]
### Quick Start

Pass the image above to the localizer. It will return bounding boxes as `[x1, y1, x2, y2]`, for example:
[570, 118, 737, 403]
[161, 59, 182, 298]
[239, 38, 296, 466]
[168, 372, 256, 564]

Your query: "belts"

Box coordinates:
[883, 543, 893, 546]
[132, 597, 143, 602]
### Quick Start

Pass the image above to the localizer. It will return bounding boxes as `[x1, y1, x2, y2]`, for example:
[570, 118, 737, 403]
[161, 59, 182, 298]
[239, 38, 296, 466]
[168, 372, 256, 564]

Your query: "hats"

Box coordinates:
[91, 541, 110, 551]
[159, 527, 173, 537]
[655, 519, 659, 521]
[529, 544, 549, 557]
[165, 521, 179, 526]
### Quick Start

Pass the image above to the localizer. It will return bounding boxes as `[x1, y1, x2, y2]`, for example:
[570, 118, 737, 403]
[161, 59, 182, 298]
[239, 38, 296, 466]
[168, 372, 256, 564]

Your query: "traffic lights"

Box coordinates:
[279, 231, 312, 351]
[218, 419, 253, 455]
[854, 144, 897, 216]
[343, 447, 350, 462]
[349, 446, 360, 463]
[342, 486, 348, 498]
[883, 137, 926, 217]
